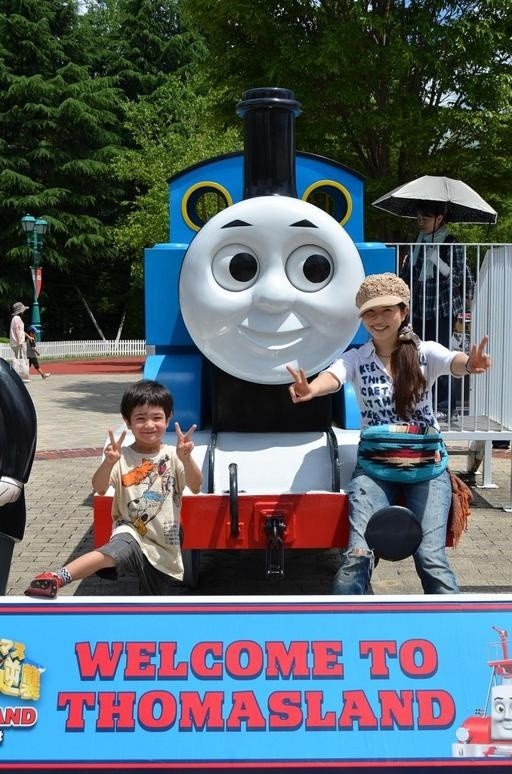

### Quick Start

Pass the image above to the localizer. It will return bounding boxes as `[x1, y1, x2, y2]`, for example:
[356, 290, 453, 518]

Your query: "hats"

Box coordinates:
[29, 327, 40, 333]
[355, 272, 410, 319]
[11, 302, 29, 315]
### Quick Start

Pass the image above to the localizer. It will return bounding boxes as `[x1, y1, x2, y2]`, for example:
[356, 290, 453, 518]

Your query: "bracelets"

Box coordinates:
[466, 357, 473, 376]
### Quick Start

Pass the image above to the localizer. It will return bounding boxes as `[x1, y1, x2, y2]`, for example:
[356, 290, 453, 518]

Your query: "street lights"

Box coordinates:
[22, 212, 50, 342]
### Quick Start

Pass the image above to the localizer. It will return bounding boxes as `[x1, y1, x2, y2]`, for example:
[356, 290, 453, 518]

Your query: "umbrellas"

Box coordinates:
[372, 174, 498, 248]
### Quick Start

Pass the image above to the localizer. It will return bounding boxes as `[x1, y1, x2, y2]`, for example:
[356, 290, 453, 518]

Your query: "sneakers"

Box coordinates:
[24, 572, 62, 597]
[42, 372, 51, 378]
[22, 379, 31, 383]
[433, 411, 460, 422]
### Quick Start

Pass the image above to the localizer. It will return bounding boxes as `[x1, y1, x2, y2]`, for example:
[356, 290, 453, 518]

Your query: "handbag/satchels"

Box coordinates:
[10, 349, 29, 379]
[448, 472, 474, 549]
[356, 425, 448, 484]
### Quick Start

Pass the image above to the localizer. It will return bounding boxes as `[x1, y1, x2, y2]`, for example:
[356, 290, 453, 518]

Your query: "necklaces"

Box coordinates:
[375, 351, 391, 357]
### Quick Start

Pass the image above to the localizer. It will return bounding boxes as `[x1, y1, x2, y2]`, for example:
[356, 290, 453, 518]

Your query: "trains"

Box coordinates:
[91, 87, 423, 592]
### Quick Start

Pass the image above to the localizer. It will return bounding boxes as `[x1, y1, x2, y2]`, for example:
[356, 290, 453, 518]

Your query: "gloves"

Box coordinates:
[427, 247, 450, 276]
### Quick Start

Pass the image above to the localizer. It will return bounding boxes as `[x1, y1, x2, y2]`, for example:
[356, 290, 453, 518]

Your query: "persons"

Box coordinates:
[24, 325, 51, 379]
[399, 198, 474, 423]
[0, 357, 37, 596]
[284, 272, 493, 595]
[27, 379, 205, 596]
[8, 301, 35, 383]
